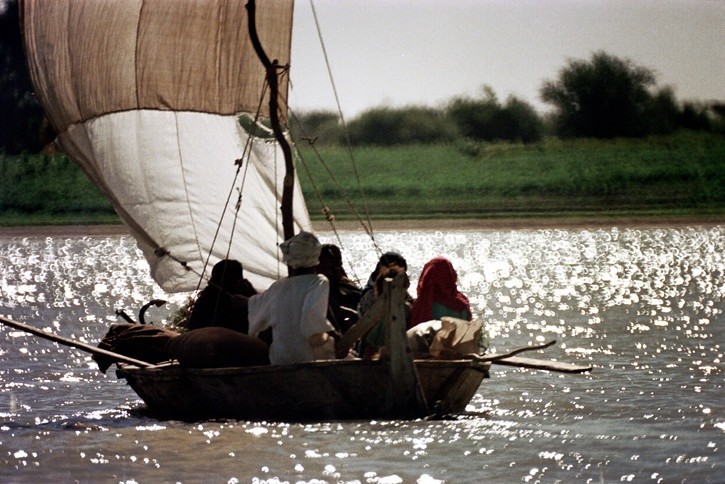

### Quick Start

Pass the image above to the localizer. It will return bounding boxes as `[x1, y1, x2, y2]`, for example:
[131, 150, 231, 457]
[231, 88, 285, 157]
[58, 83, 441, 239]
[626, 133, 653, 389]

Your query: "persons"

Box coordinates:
[319, 244, 361, 334]
[344, 264, 415, 363]
[359, 252, 408, 315]
[188, 258, 272, 348]
[408, 257, 472, 329]
[230, 231, 354, 366]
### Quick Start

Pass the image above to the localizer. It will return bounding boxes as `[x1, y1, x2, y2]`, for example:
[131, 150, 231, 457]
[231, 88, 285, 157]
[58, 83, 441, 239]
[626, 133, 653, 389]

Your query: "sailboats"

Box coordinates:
[0, 0, 593, 423]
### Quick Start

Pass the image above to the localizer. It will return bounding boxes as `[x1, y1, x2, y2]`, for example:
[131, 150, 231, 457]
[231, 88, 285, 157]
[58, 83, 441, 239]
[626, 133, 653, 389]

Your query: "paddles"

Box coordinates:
[489, 357, 593, 374]
[459, 340, 557, 362]
[0, 316, 154, 368]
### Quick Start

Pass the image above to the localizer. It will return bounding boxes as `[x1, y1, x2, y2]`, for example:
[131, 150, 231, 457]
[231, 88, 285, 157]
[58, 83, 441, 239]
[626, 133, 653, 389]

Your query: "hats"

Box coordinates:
[279, 232, 322, 270]
